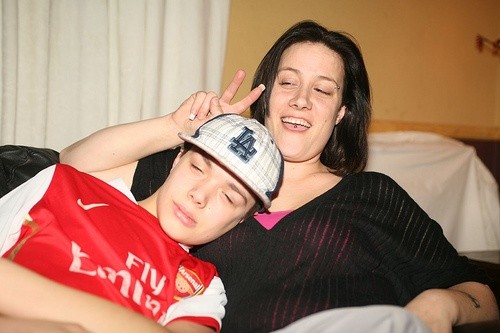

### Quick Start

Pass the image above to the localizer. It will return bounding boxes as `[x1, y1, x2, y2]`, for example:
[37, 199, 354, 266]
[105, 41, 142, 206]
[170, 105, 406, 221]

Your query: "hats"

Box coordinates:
[178, 113, 282, 213]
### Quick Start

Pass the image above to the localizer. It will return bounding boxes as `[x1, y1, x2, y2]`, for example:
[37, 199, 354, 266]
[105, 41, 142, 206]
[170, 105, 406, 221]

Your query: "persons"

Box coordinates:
[58, 18, 500, 333]
[0, 113, 283, 332]
